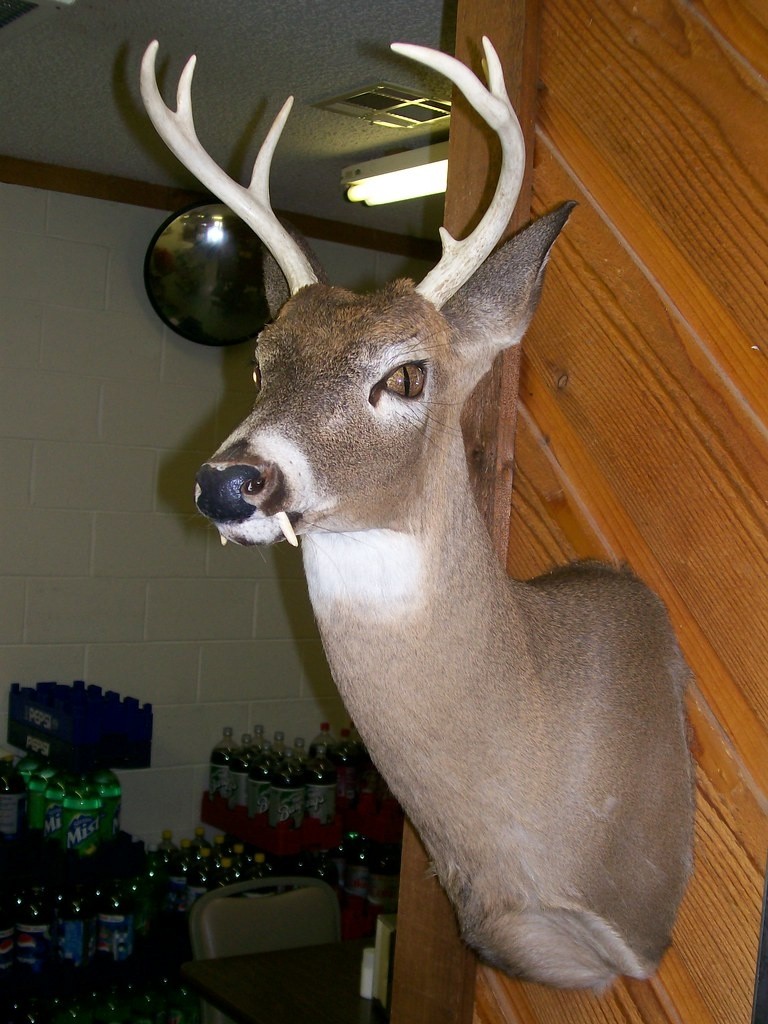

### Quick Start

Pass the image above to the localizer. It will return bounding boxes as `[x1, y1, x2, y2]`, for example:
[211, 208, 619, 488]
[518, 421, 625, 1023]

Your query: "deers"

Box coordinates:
[138, 36, 695, 995]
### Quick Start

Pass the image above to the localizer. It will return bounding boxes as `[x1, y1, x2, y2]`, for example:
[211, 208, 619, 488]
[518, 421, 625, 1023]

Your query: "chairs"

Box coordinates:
[190, 876, 342, 1024]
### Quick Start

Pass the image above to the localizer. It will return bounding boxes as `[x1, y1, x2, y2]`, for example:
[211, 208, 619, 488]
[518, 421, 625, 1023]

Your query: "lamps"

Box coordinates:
[339, 141, 447, 209]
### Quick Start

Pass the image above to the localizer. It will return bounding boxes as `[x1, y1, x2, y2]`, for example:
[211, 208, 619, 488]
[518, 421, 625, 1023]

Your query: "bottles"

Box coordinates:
[0, 721, 400, 1024]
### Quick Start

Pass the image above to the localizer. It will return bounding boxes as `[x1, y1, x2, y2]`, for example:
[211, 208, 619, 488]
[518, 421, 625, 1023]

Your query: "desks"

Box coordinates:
[180, 935, 390, 1024]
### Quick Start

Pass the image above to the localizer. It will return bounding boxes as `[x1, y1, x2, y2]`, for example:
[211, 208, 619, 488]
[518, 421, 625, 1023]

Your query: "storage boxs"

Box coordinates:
[337, 793, 404, 844]
[6, 680, 154, 780]
[0, 830, 144, 885]
[0, 952, 143, 1002]
[200, 790, 343, 856]
